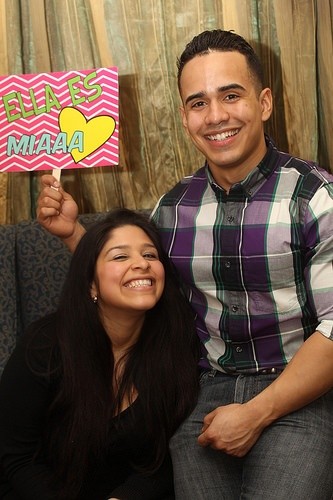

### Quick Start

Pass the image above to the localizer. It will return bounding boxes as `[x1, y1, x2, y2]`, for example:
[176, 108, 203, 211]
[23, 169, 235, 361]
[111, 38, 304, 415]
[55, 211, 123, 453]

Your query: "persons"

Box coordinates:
[35, 29, 333, 500]
[0, 206, 203, 500]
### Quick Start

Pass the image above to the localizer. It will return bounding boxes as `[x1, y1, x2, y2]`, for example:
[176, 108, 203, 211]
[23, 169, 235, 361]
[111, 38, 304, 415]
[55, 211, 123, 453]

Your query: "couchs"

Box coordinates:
[0, 209, 155, 380]
